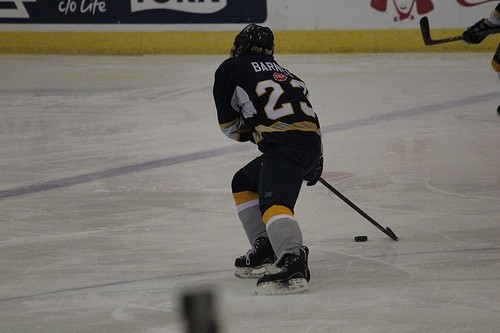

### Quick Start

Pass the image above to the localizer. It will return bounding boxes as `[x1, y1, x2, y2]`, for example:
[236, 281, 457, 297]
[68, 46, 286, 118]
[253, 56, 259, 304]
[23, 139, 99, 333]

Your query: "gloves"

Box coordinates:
[304, 154, 324, 186]
[463, 18, 493, 44]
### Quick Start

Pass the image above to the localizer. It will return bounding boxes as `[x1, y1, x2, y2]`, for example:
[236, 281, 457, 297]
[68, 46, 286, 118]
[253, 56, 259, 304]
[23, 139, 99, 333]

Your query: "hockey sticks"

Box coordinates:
[419, 16, 500, 46]
[318, 176, 399, 241]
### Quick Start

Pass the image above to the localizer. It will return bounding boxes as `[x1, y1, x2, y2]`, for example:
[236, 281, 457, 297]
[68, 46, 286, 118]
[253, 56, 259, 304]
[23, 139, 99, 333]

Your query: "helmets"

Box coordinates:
[230, 24, 276, 59]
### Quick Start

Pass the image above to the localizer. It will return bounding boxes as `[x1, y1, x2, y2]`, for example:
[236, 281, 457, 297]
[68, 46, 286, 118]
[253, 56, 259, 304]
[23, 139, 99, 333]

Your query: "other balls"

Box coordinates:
[354, 235, 368, 242]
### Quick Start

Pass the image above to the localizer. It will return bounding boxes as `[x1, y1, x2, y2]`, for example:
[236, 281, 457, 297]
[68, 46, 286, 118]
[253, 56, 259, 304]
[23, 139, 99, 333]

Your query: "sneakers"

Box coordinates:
[256, 246, 311, 295]
[235, 237, 277, 279]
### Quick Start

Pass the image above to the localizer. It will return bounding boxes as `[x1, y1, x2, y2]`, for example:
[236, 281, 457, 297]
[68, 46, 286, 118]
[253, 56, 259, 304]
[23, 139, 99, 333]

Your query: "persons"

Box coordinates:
[212, 23, 324, 295]
[462, 2, 500, 115]
